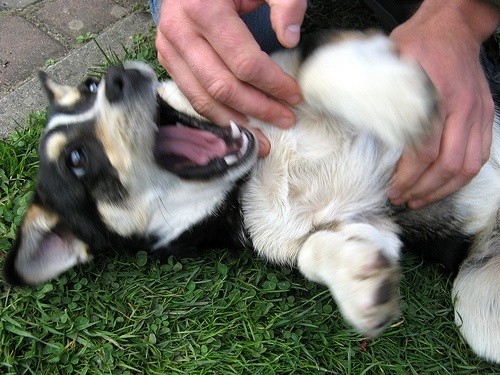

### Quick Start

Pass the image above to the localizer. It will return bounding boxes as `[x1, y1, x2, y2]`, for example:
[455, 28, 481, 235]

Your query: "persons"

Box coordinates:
[150, 0, 500, 209]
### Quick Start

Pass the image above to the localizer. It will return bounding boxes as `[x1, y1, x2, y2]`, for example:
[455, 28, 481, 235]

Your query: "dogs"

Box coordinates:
[4, 31, 500, 365]
